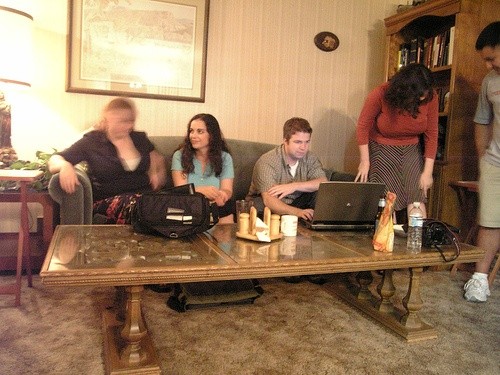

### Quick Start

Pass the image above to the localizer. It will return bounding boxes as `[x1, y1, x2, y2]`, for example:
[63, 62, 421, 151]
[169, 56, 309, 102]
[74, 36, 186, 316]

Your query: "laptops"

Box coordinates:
[300, 182, 386, 230]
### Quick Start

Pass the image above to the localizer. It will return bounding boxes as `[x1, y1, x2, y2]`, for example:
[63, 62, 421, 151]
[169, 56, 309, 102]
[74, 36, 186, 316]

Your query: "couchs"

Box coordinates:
[48, 136, 361, 225]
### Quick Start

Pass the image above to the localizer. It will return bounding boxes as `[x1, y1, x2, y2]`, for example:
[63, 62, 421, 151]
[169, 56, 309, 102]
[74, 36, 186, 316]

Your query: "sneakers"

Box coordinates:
[464, 272, 491, 303]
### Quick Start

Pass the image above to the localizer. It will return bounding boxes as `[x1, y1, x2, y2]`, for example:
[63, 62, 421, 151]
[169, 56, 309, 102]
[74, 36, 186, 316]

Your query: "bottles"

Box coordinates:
[374, 194, 385, 232]
[407, 202, 423, 249]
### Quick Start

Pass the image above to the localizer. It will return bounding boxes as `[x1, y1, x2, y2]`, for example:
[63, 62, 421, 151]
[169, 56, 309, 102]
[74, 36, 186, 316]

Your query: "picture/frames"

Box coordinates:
[65, 0, 210, 103]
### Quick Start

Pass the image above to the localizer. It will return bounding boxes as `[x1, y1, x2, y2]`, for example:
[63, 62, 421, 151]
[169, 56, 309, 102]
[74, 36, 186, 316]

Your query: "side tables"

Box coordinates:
[0, 189, 58, 272]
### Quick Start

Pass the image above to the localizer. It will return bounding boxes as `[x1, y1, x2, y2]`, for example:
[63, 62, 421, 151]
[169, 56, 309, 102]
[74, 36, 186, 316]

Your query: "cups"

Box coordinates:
[281, 215, 298, 236]
[236, 200, 253, 232]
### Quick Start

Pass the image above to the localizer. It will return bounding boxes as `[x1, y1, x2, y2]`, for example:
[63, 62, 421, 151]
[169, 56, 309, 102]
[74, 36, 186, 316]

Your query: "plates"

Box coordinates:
[393, 224, 407, 238]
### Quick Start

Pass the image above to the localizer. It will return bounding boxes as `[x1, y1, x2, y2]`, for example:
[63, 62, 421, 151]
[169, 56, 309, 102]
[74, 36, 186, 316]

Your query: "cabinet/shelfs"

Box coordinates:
[384, 0, 500, 272]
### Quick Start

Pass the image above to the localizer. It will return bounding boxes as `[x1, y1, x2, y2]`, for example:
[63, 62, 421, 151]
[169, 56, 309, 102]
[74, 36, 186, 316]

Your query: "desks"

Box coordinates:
[448, 180, 500, 284]
[0, 169, 44, 308]
[40, 224, 486, 375]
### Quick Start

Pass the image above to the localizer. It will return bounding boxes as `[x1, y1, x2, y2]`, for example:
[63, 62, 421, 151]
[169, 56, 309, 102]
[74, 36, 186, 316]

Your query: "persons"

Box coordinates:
[47, 98, 166, 222]
[464, 22, 500, 302]
[355, 63, 438, 224]
[245, 117, 329, 283]
[171, 113, 237, 221]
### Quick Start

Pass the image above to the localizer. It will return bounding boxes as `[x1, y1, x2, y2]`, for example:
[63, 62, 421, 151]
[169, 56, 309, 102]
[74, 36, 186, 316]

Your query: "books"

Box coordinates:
[436, 88, 449, 112]
[398, 27, 456, 69]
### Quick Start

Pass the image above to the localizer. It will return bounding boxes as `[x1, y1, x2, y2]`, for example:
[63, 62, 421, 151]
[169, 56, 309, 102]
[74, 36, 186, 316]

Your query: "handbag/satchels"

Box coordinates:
[129, 183, 220, 239]
[402, 219, 460, 246]
